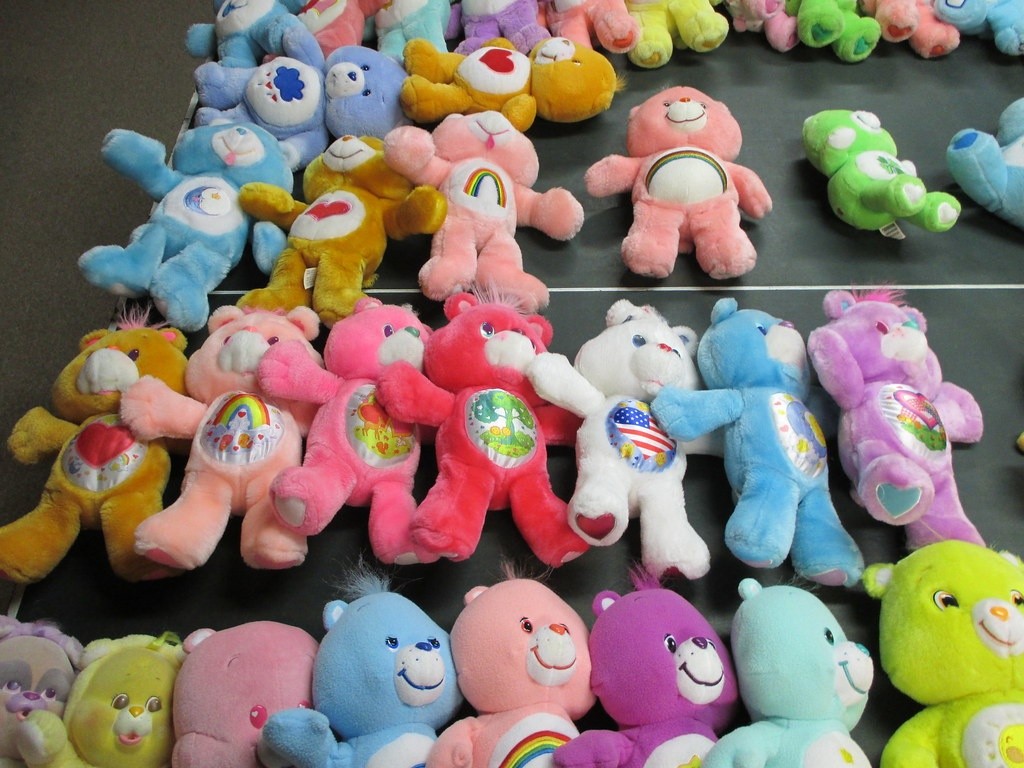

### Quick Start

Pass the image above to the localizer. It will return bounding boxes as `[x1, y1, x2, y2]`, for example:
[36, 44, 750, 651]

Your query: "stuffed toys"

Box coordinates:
[383, 110, 585, 311]
[584, 85, 773, 280]
[801, 109, 962, 240]
[237, 135, 448, 329]
[400, 36, 623, 131]
[119, 304, 325, 569]
[0, 301, 190, 586]
[553, 559, 738, 768]
[946, 96, 1024, 227]
[524, 298, 724, 580]
[649, 296, 865, 588]
[192, 44, 413, 173]
[807, 278, 990, 552]
[262, 549, 466, 768]
[256, 297, 442, 565]
[375, 277, 591, 567]
[0, 614, 84, 768]
[171, 620, 320, 768]
[15, 631, 188, 768]
[74, 117, 299, 336]
[425, 554, 597, 768]
[699, 571, 875, 768]
[861, 539, 1024, 768]
[181, 0, 1024, 69]
